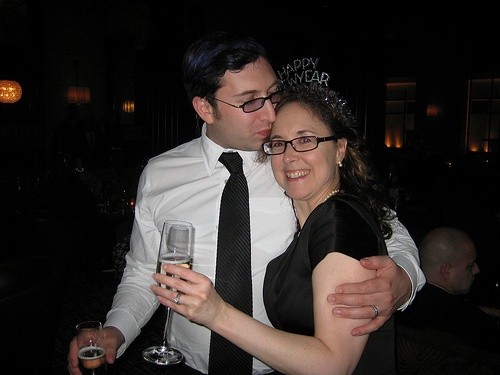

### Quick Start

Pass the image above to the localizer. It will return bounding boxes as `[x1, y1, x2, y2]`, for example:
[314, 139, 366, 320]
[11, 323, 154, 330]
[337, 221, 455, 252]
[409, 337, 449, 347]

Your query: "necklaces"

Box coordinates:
[297, 189, 339, 237]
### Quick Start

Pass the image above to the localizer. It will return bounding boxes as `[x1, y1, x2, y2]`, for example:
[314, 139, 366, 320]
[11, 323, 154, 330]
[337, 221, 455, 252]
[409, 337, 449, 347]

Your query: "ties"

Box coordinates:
[207, 152, 254, 375]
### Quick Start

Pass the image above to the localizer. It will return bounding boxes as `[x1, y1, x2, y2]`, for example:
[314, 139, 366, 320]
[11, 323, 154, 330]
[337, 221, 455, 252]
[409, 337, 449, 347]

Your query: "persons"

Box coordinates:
[68, 32, 426, 375]
[395, 228, 500, 351]
[151, 92, 395, 375]
[51, 102, 129, 166]
[401, 130, 416, 160]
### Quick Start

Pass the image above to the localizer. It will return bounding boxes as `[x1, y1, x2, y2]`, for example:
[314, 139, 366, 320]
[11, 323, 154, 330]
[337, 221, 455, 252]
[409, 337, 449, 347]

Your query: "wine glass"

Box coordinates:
[143, 220, 193, 366]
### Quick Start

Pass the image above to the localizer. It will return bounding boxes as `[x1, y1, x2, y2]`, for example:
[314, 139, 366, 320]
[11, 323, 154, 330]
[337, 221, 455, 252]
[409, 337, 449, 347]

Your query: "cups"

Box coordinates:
[76, 321, 107, 375]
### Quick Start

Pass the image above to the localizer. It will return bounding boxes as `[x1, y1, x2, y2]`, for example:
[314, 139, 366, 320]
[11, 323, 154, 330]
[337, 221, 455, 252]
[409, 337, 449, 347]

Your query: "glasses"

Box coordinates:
[262, 136, 340, 156]
[206, 81, 286, 113]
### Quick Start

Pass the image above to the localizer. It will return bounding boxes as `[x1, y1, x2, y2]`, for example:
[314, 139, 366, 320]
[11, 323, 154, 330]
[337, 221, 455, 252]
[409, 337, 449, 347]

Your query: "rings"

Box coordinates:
[174, 293, 182, 305]
[370, 305, 378, 319]
